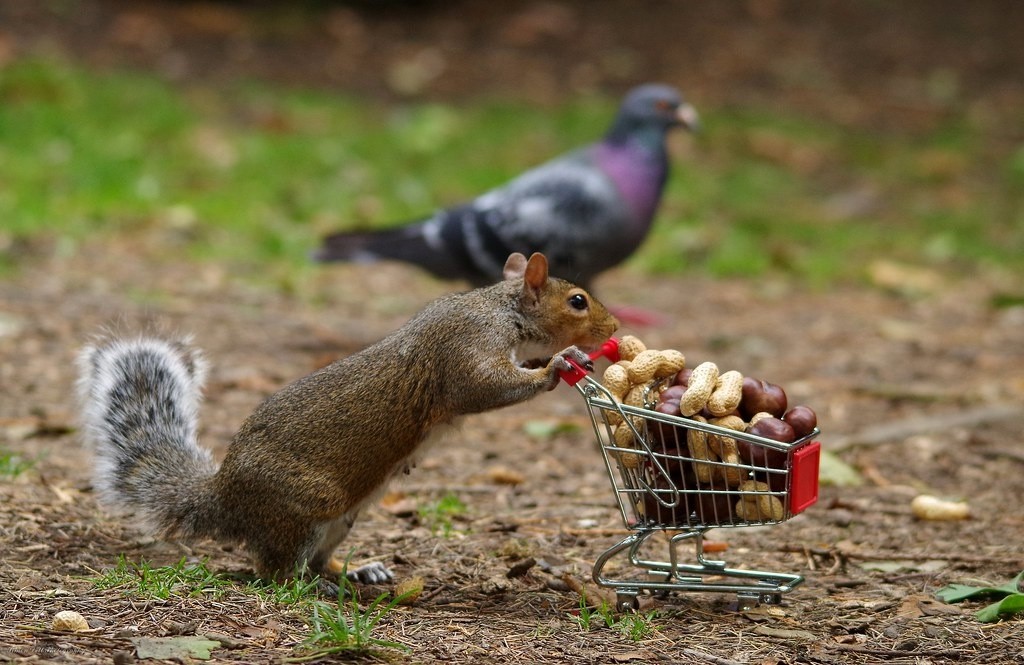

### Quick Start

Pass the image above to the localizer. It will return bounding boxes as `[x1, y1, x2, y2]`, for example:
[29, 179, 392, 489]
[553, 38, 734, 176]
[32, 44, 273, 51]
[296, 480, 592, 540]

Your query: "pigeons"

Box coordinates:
[310, 83, 701, 326]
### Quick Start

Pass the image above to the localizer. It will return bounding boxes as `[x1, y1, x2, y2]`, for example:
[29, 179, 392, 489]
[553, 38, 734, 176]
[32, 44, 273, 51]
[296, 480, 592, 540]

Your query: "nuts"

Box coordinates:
[394, 574, 425, 603]
[911, 496, 972, 519]
[598, 333, 785, 521]
[52, 610, 90, 631]
[491, 466, 522, 483]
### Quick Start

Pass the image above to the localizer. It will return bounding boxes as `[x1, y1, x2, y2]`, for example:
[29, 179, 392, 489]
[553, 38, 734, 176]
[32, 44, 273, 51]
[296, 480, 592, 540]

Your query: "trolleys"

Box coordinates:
[558, 337, 822, 614]
